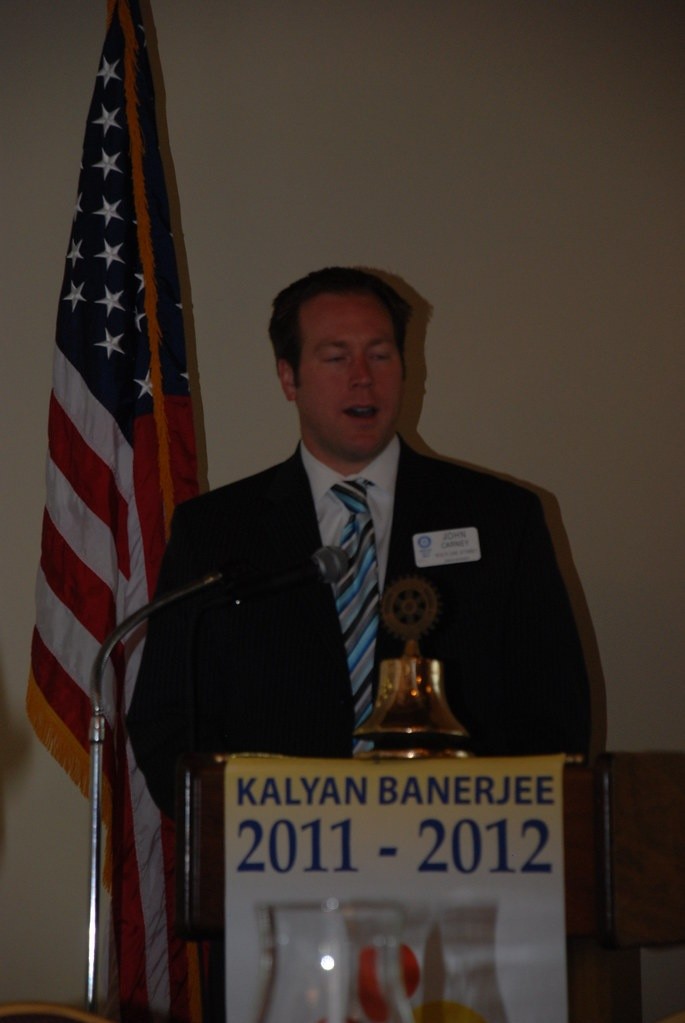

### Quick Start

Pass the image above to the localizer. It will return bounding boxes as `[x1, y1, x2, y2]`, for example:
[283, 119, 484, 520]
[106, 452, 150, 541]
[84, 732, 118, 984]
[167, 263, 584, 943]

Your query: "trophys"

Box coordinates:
[350, 576, 481, 764]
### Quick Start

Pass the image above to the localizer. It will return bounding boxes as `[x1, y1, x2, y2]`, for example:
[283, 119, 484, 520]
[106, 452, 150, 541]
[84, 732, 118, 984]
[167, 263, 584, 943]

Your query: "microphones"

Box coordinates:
[230, 545, 351, 610]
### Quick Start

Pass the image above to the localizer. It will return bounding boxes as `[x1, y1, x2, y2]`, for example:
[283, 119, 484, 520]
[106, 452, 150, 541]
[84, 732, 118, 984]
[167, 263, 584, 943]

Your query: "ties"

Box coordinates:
[326, 477, 383, 755]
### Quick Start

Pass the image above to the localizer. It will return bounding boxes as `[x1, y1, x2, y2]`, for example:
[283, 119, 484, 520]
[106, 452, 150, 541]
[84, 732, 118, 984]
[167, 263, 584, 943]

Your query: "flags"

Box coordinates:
[23, 2, 227, 1021]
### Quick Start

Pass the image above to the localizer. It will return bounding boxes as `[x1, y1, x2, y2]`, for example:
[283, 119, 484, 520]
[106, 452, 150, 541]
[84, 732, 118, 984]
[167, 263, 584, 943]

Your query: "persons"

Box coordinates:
[129, 267, 610, 834]
